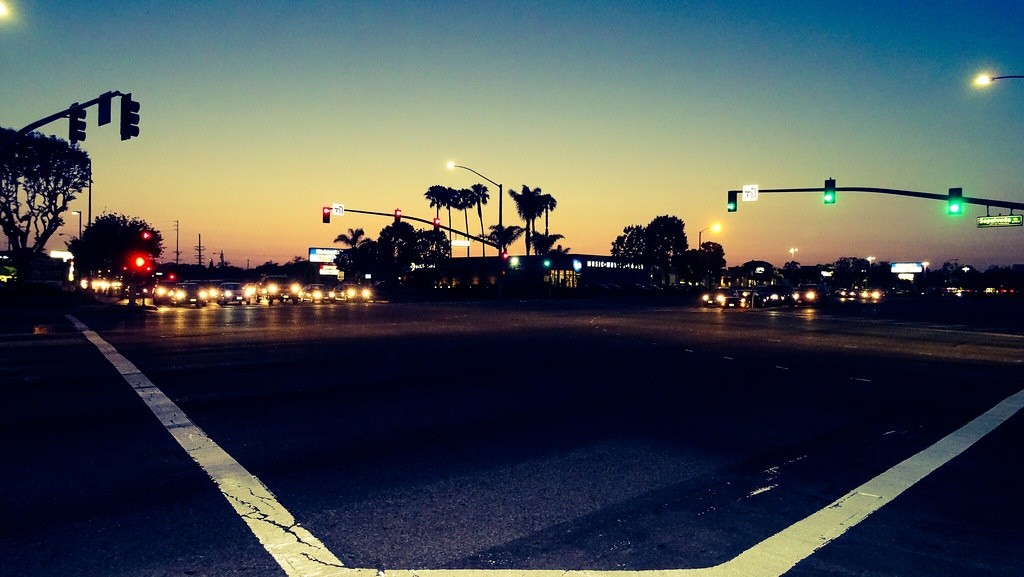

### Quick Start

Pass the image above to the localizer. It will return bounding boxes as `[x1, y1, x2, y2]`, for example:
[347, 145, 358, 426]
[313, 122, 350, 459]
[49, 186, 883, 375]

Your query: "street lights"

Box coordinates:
[448, 163, 502, 256]
[73, 211, 82, 239]
[213, 253, 223, 263]
[699, 226, 718, 249]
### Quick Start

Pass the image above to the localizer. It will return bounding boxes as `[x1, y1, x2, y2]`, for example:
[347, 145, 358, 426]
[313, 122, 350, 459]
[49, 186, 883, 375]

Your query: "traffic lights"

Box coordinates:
[948, 188, 963, 215]
[434, 218, 440, 231]
[69, 102, 86, 144]
[824, 179, 836, 204]
[135, 258, 145, 267]
[544, 260, 551, 268]
[728, 191, 737, 212]
[323, 207, 331, 223]
[120, 93, 140, 141]
[395, 210, 401, 223]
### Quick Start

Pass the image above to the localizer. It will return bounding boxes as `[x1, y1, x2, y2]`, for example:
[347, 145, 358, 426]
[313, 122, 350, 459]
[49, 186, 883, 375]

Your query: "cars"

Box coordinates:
[152, 281, 176, 306]
[738, 284, 884, 308]
[125, 284, 147, 299]
[185, 275, 389, 312]
[703, 287, 740, 308]
[170, 282, 207, 308]
